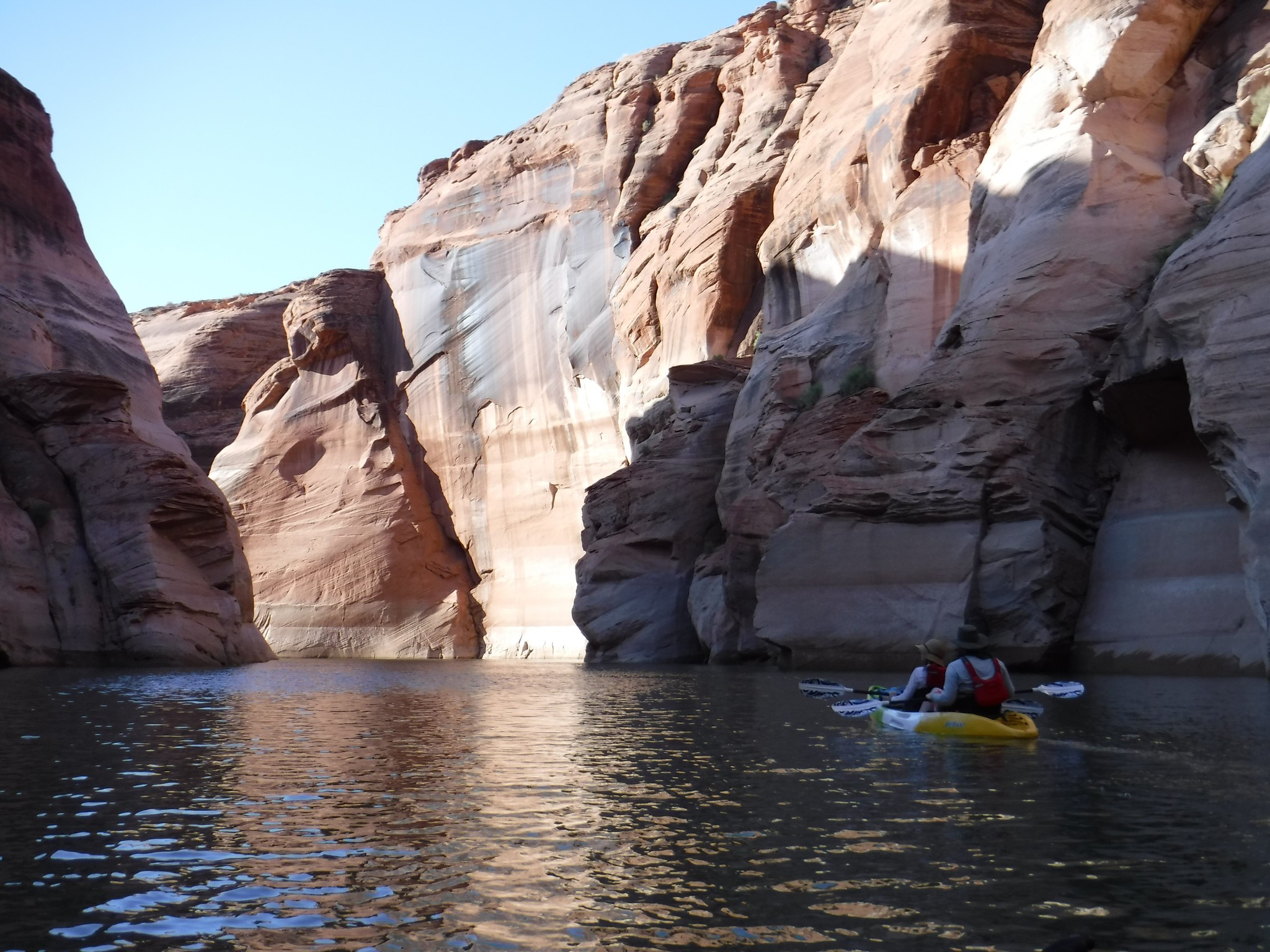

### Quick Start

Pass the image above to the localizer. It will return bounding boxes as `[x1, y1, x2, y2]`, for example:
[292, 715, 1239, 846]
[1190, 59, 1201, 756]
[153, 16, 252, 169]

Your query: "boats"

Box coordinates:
[867, 700, 1038, 739]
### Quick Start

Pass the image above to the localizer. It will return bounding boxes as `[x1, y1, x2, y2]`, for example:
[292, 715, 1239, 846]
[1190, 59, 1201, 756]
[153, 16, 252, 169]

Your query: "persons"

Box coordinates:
[888, 624, 1015, 720]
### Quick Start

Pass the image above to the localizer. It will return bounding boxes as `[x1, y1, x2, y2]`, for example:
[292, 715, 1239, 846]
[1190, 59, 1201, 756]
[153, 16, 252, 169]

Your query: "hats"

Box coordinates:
[949, 624, 989, 649]
[915, 638, 947, 667]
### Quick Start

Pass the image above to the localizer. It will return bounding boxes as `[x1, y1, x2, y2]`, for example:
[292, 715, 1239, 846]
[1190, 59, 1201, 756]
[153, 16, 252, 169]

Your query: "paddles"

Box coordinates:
[833, 679, 1084, 718]
[797, 675, 1047, 719]
